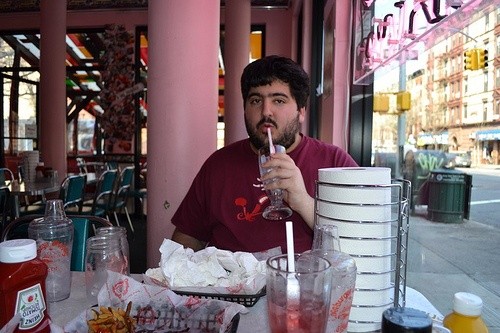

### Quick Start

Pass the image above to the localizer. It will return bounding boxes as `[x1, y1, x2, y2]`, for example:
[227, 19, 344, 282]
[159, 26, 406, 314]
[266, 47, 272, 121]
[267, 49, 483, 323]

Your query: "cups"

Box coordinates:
[428, 325, 451, 333]
[266, 252, 332, 333]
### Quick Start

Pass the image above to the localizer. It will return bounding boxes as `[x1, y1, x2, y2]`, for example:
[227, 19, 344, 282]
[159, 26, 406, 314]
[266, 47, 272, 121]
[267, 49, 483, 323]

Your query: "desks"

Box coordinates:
[0, 173, 446, 333]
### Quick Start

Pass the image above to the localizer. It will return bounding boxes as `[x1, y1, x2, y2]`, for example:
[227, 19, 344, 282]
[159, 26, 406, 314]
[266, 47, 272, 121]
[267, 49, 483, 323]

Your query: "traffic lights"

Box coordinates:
[464, 48, 476, 71]
[475, 48, 489, 71]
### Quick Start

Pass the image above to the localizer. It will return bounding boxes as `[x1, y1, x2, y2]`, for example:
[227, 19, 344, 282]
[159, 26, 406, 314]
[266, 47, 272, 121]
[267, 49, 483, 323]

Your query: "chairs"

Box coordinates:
[0, 164, 148, 275]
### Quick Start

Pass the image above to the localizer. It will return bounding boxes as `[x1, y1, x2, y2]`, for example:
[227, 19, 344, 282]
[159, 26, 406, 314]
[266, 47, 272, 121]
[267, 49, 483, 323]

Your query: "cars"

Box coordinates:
[453, 150, 472, 168]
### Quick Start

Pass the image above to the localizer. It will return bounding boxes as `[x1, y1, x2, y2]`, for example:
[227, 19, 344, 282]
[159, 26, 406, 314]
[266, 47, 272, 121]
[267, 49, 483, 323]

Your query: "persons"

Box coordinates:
[170, 55, 361, 255]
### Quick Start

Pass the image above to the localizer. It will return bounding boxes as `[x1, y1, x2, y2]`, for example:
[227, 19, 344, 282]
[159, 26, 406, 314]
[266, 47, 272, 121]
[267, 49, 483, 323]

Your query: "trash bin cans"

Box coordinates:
[427, 169, 473, 223]
[373, 151, 397, 178]
[404, 150, 456, 216]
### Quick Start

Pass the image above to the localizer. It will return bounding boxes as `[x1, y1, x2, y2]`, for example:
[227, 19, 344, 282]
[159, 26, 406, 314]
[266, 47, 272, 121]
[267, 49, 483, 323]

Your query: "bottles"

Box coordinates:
[84, 234, 128, 305]
[0, 238, 51, 333]
[27, 199, 73, 303]
[444, 291, 491, 333]
[381, 306, 433, 333]
[96, 226, 129, 277]
[294, 226, 357, 333]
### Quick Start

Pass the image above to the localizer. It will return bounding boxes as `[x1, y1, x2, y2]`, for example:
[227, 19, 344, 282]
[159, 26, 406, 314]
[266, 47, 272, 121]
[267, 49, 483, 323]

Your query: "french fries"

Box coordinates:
[88, 301, 133, 333]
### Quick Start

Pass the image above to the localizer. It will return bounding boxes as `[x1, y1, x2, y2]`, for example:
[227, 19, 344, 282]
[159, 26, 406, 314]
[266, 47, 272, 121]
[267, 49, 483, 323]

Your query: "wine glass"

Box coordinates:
[258, 144, 293, 221]
